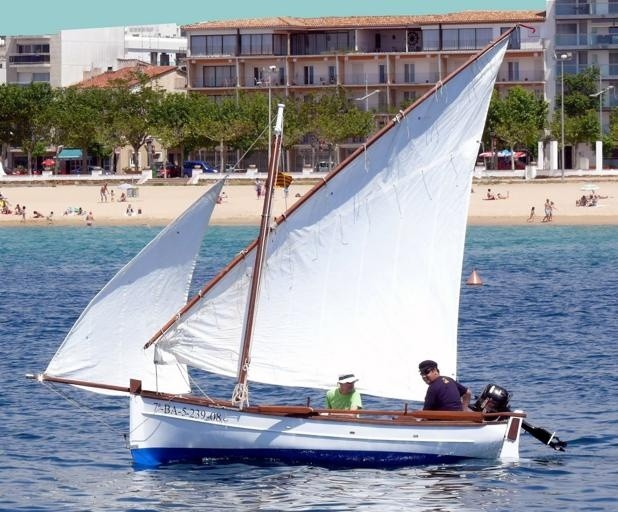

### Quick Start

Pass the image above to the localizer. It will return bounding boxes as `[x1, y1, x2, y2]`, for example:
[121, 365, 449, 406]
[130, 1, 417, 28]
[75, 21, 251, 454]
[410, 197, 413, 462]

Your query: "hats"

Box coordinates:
[337, 372, 359, 384]
[419, 360, 437, 370]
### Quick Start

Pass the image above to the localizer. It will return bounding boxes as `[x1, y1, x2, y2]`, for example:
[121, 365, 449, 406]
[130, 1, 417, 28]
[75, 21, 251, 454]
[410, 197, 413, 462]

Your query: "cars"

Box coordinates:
[72, 166, 108, 175]
[156, 160, 247, 178]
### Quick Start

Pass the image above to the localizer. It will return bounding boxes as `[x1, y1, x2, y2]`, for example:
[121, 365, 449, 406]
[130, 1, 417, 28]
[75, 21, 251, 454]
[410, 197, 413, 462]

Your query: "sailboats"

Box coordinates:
[25, 22, 568, 473]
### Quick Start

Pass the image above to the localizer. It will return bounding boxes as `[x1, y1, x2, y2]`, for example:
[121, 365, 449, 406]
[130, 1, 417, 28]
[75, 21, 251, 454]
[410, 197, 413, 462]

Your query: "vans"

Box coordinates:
[315, 161, 337, 172]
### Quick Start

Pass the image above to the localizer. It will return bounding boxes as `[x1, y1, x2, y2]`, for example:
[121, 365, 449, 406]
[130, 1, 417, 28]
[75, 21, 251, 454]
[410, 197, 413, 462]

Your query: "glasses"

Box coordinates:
[420, 371, 430, 376]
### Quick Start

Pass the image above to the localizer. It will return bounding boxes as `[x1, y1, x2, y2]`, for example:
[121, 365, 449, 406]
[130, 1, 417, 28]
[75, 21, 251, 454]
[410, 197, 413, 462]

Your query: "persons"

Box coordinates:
[77, 207, 83, 216]
[326, 373, 362, 418]
[0, 192, 27, 224]
[117, 192, 126, 202]
[217, 192, 227, 203]
[255, 178, 264, 200]
[419, 360, 471, 421]
[543, 199, 554, 222]
[126, 204, 133, 216]
[487, 188, 509, 200]
[504, 156, 510, 169]
[100, 182, 116, 202]
[527, 207, 535, 222]
[33, 211, 44, 218]
[86, 211, 95, 225]
[576, 189, 608, 207]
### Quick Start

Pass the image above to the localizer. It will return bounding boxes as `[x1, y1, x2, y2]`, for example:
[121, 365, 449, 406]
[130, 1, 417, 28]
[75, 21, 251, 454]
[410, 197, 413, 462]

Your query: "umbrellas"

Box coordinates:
[479, 152, 496, 162]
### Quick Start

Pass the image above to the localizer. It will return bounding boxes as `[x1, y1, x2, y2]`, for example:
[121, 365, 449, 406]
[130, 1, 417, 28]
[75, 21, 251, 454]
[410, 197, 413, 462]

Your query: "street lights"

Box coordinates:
[354, 72, 380, 112]
[552, 52, 573, 184]
[589, 70, 614, 136]
